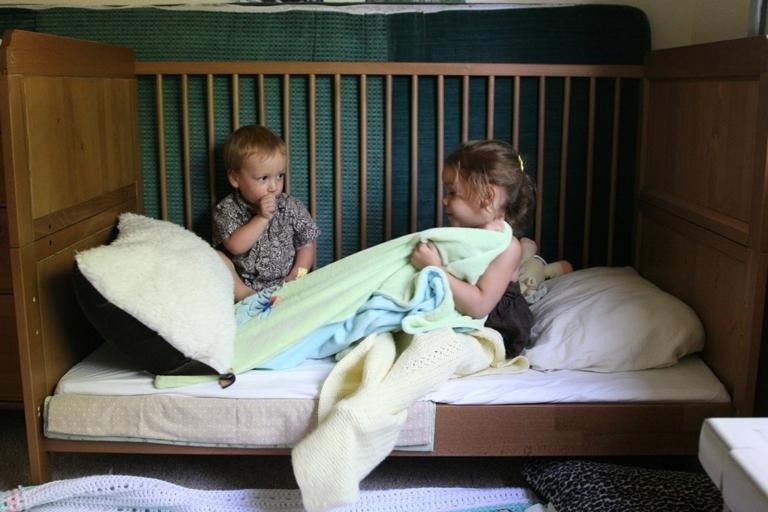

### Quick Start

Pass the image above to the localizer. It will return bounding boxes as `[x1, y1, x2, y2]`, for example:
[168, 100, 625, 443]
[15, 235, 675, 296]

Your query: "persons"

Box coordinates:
[410, 139, 537, 360]
[210, 123, 321, 301]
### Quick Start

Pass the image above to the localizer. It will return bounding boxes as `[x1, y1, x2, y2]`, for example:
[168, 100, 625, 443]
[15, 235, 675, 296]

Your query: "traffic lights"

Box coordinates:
[521, 265, 707, 373]
[520, 451, 723, 511]
[74, 212, 235, 376]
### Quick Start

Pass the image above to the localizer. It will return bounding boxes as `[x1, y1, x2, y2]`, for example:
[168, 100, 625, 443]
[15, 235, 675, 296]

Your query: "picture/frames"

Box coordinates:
[698, 417, 767, 511]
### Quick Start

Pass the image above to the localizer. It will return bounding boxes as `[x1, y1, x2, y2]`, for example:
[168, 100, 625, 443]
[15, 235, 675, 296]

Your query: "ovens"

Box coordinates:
[0, 27, 768, 486]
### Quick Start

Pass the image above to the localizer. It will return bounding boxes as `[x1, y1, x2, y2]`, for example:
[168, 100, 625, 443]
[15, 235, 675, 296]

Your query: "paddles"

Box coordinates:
[0, 474, 544, 511]
